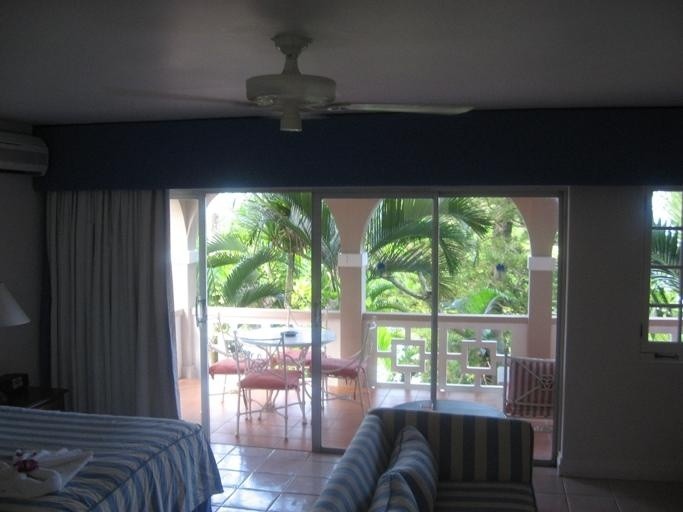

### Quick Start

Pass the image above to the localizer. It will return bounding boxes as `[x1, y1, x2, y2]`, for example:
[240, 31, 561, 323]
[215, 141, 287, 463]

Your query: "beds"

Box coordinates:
[0, 405, 203, 512]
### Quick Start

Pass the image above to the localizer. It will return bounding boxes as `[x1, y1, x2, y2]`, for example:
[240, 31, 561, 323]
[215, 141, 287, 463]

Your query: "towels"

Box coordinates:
[506, 357, 555, 420]
[0, 447, 93, 499]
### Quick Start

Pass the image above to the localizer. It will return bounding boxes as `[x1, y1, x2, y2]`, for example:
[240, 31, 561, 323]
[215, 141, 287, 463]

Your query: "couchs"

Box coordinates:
[311, 407, 537, 512]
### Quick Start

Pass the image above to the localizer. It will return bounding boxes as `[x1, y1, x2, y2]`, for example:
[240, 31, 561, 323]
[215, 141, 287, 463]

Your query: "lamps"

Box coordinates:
[0, 283, 31, 329]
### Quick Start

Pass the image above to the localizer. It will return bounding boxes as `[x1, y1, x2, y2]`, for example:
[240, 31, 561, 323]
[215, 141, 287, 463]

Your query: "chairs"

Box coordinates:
[208, 313, 372, 443]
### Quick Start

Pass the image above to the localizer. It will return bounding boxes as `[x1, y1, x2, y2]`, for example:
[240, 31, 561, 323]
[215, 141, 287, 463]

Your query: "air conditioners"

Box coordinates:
[1, 131, 49, 177]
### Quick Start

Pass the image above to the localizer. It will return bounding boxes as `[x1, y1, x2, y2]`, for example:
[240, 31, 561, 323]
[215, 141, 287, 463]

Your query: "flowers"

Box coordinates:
[14, 459, 38, 471]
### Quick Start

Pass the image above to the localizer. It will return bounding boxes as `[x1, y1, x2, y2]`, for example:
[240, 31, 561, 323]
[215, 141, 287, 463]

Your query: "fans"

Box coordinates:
[107, 34, 478, 134]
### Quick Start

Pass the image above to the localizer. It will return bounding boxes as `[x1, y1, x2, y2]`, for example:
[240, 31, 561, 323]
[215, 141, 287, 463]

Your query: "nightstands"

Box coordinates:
[0, 386, 69, 410]
